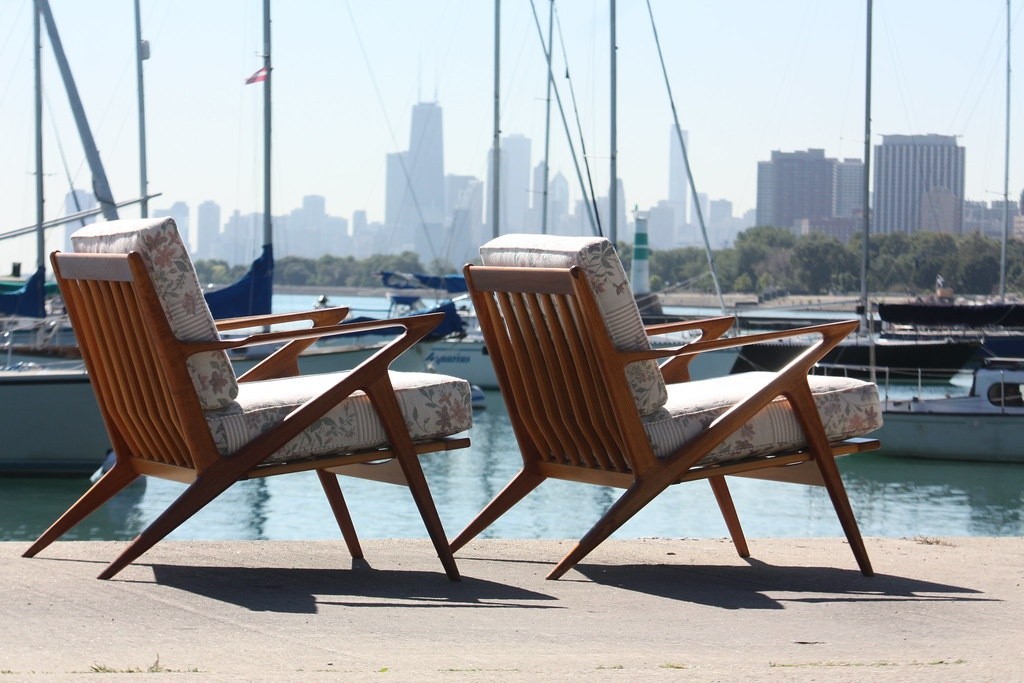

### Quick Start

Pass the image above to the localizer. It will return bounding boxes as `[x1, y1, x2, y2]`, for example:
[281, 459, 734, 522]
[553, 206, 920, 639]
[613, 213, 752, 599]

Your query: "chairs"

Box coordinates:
[449, 234, 884, 581]
[23, 217, 473, 579]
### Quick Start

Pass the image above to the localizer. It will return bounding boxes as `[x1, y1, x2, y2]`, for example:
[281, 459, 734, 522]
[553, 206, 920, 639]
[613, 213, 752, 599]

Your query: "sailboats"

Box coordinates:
[1, 0, 1024, 476]
